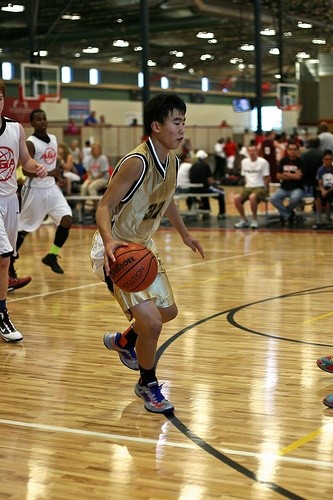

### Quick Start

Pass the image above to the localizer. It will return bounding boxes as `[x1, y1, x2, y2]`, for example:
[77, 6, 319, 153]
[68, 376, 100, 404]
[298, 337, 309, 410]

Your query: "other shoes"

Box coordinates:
[218, 215, 226, 220]
[202, 215, 210, 221]
[325, 223, 332, 229]
[312, 223, 322, 230]
[279, 211, 295, 222]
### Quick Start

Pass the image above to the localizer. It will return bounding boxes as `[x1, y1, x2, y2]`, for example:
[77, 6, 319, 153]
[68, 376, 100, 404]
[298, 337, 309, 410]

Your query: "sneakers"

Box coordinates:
[42, 253, 64, 274]
[135, 377, 174, 413]
[103, 332, 140, 371]
[0, 309, 23, 342]
[317, 356, 333, 373]
[251, 220, 258, 229]
[234, 219, 249, 228]
[323, 394, 333, 407]
[8, 261, 17, 279]
[7, 270, 31, 292]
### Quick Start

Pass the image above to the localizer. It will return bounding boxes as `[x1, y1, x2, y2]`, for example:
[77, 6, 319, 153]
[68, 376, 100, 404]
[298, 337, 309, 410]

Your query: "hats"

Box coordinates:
[196, 150, 208, 160]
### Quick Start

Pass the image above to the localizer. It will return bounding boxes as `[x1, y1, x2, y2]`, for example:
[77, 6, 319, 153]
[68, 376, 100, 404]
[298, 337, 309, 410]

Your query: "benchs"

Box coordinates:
[64, 181, 333, 225]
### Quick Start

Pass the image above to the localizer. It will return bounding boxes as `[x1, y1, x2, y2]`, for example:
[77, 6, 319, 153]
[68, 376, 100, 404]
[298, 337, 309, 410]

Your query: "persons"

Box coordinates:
[317, 356, 332, 408]
[179, 119, 333, 197]
[8, 108, 72, 279]
[312, 151, 333, 229]
[89, 93, 204, 413]
[233, 144, 270, 228]
[177, 153, 202, 218]
[7, 264, 31, 292]
[270, 142, 307, 223]
[188, 150, 231, 219]
[1, 83, 47, 343]
[55, 111, 140, 220]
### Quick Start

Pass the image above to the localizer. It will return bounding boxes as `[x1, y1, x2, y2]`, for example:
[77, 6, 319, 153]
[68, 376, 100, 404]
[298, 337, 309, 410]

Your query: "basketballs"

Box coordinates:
[109, 243, 158, 293]
